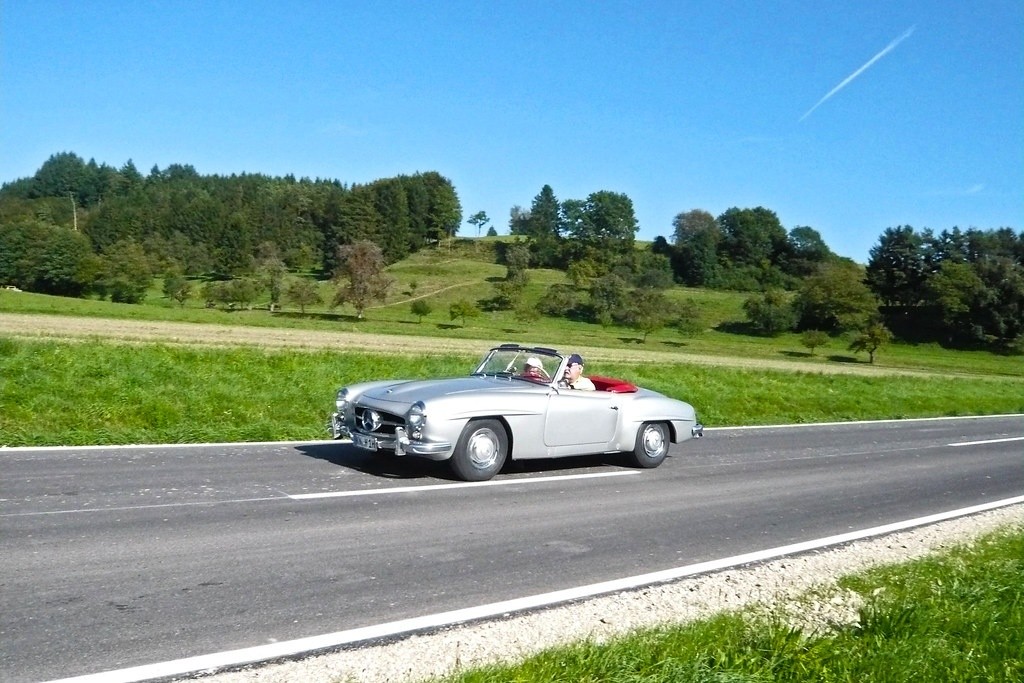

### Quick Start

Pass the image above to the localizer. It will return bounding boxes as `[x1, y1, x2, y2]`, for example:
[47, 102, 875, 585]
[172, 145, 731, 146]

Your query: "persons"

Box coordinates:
[559, 354, 595, 391]
[523, 357, 543, 379]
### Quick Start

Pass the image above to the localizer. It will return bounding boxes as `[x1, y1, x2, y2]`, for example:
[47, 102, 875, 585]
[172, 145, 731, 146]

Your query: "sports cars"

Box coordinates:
[330, 342, 704, 483]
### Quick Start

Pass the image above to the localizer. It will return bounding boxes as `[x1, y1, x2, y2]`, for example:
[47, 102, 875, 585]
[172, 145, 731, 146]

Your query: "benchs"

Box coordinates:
[586, 375, 637, 393]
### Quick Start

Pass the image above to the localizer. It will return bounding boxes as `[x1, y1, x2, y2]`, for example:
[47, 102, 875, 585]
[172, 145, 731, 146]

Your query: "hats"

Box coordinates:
[561, 354, 586, 365]
[525, 356, 544, 369]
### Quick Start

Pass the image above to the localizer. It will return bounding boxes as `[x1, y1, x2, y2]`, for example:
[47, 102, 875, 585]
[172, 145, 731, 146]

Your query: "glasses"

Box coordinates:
[567, 364, 573, 368]
